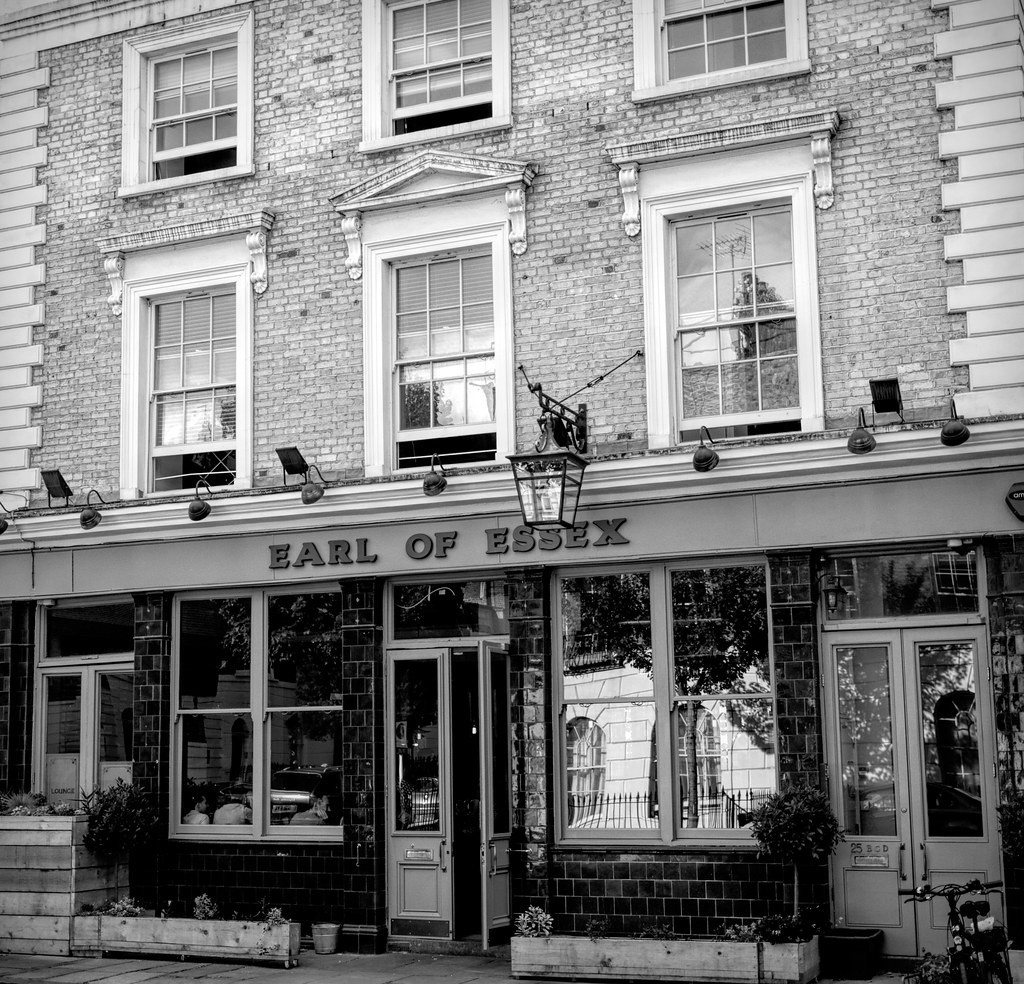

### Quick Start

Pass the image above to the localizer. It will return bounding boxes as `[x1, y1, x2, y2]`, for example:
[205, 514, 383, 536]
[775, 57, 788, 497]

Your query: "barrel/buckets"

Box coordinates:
[311, 923, 340, 954]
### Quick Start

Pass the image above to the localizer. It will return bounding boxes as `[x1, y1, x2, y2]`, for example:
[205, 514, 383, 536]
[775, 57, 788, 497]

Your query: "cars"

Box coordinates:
[413, 776, 439, 830]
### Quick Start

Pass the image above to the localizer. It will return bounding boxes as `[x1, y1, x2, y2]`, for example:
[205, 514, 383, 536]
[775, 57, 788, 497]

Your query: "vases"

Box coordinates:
[311, 923, 342, 954]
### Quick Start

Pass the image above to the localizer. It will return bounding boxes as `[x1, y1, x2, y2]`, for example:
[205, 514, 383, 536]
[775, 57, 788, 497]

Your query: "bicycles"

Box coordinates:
[897, 878, 1012, 984]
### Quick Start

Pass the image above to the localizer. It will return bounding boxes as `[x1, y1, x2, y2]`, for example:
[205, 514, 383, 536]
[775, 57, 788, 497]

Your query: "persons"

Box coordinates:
[183, 786, 252, 825]
[290, 789, 332, 824]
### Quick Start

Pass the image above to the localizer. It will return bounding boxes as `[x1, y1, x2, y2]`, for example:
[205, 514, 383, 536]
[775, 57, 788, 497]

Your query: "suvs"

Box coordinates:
[270, 765, 342, 826]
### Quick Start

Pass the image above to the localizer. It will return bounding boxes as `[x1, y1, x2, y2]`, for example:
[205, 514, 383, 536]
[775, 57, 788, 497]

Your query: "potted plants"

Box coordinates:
[511, 785, 847, 984]
[71, 778, 301, 967]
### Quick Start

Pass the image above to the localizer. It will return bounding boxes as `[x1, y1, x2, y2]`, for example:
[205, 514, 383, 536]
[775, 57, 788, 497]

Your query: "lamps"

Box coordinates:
[507, 382, 590, 532]
[941, 399, 970, 446]
[40, 470, 73, 509]
[692, 426, 719, 472]
[423, 453, 456, 496]
[80, 490, 121, 530]
[275, 446, 309, 486]
[847, 407, 876, 455]
[188, 480, 211, 521]
[301, 465, 336, 505]
[869, 377, 905, 432]
